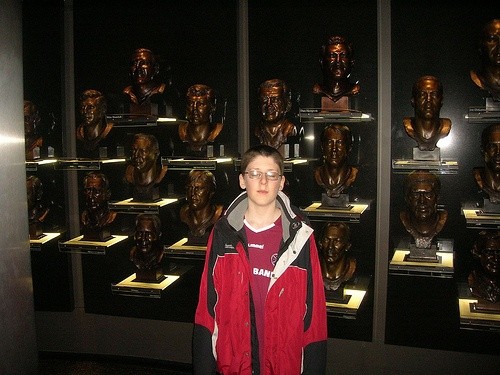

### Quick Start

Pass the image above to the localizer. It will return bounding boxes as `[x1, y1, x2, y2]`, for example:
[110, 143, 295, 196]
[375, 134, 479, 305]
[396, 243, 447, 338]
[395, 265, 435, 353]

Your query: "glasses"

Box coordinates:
[244, 170, 282, 181]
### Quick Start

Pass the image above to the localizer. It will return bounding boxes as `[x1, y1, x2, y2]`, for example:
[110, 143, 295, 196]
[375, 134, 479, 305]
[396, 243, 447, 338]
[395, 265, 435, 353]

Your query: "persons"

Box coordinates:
[191, 143, 328, 375]
[24, 19, 500, 313]
